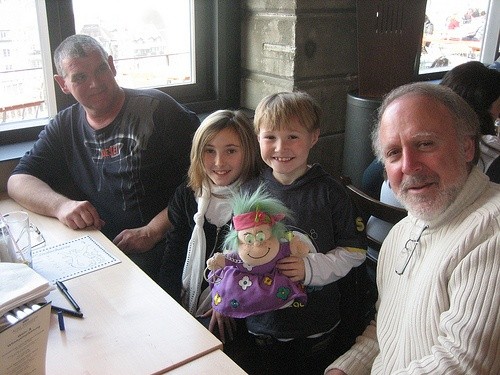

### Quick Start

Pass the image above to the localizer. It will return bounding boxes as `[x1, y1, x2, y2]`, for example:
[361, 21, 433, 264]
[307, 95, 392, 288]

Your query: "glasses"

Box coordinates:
[395, 224, 429, 275]
[16, 222, 45, 253]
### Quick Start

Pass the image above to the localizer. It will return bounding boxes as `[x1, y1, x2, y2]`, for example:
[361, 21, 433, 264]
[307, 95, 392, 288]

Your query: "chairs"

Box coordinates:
[340, 175, 408, 326]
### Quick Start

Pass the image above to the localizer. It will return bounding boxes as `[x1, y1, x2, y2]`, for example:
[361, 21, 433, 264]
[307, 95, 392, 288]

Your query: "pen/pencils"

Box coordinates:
[37, 281, 83, 331]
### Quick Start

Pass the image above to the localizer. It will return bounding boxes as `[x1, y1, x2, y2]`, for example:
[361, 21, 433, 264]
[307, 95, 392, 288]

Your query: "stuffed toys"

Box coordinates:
[207, 183, 310, 342]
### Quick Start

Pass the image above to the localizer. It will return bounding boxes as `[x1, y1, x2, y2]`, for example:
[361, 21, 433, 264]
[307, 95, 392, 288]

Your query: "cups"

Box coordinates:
[1, 211, 32, 264]
[0, 223, 18, 263]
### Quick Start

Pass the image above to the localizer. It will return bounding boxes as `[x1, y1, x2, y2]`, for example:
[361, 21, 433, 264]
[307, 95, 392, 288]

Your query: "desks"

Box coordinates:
[159, 349, 249, 375]
[0, 190, 223, 375]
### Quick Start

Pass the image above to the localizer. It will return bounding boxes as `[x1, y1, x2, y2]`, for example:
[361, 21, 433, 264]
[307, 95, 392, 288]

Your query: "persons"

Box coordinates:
[159, 109, 279, 345]
[7, 35, 201, 273]
[362, 53, 500, 289]
[321, 82, 500, 375]
[236, 92, 369, 375]
